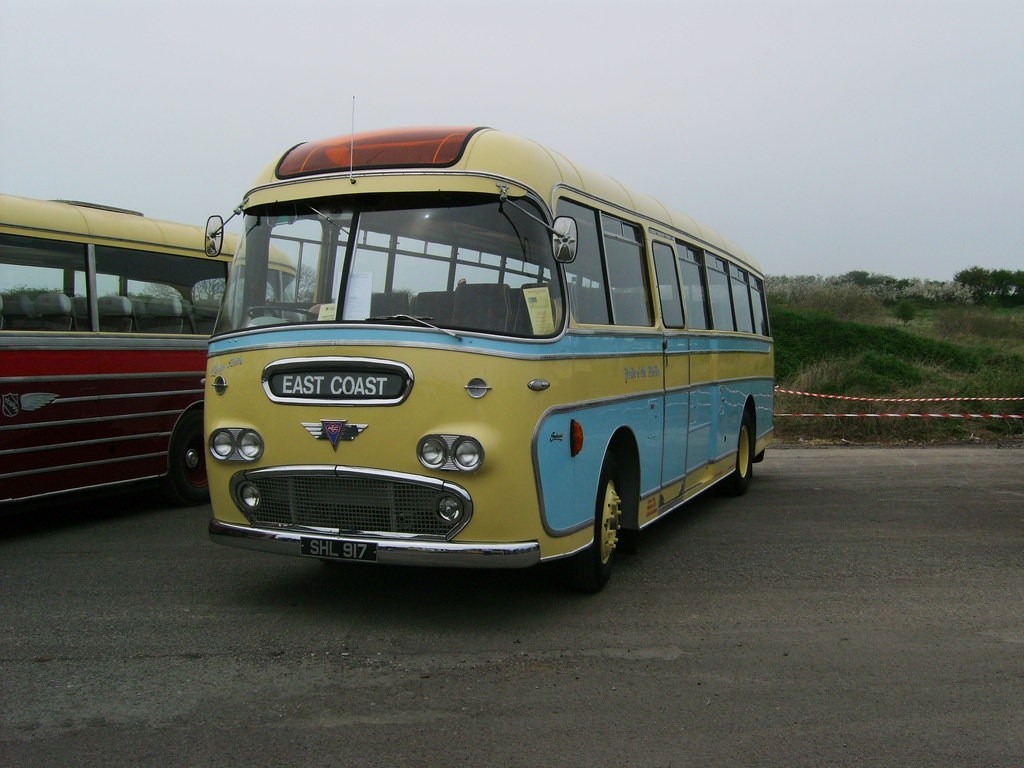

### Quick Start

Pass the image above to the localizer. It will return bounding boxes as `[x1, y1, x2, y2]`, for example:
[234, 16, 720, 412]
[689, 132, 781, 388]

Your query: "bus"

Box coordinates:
[0, 194, 299, 513]
[203, 126, 777, 596]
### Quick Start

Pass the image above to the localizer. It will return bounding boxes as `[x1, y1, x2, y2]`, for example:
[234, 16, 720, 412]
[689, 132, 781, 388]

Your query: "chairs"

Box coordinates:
[0, 293, 71, 332]
[69, 296, 132, 333]
[688, 301, 706, 329]
[131, 298, 221, 334]
[578, 287, 609, 324]
[615, 292, 647, 328]
[662, 300, 681, 326]
[370, 278, 576, 336]
